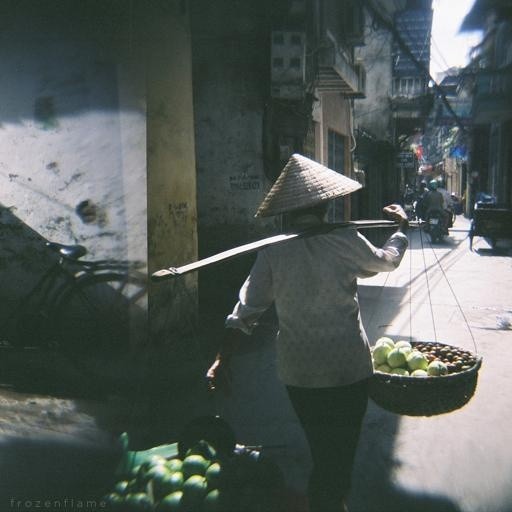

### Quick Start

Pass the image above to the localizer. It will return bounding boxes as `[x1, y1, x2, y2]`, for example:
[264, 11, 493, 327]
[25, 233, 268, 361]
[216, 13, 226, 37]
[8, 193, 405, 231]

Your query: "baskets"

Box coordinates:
[102, 438, 283, 511]
[368, 332, 482, 416]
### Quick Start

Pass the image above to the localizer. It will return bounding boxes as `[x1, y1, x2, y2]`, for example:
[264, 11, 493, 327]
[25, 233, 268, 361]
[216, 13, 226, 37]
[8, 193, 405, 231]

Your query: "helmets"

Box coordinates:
[430, 179, 438, 189]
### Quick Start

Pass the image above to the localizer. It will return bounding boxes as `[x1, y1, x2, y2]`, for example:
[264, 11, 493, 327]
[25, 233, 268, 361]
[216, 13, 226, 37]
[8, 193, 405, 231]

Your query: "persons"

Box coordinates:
[203, 152, 411, 511]
[398, 165, 466, 235]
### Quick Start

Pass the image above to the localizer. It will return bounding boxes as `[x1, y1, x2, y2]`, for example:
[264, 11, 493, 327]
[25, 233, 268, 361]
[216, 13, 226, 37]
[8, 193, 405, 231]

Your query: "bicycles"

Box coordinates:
[0, 239, 172, 390]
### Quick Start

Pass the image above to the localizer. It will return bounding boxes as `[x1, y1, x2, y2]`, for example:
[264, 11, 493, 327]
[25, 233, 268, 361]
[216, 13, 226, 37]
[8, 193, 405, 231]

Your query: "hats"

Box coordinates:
[258, 153, 365, 221]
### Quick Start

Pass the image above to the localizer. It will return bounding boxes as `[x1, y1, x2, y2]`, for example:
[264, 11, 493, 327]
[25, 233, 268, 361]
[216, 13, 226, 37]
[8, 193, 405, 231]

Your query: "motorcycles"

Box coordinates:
[424, 204, 460, 246]
